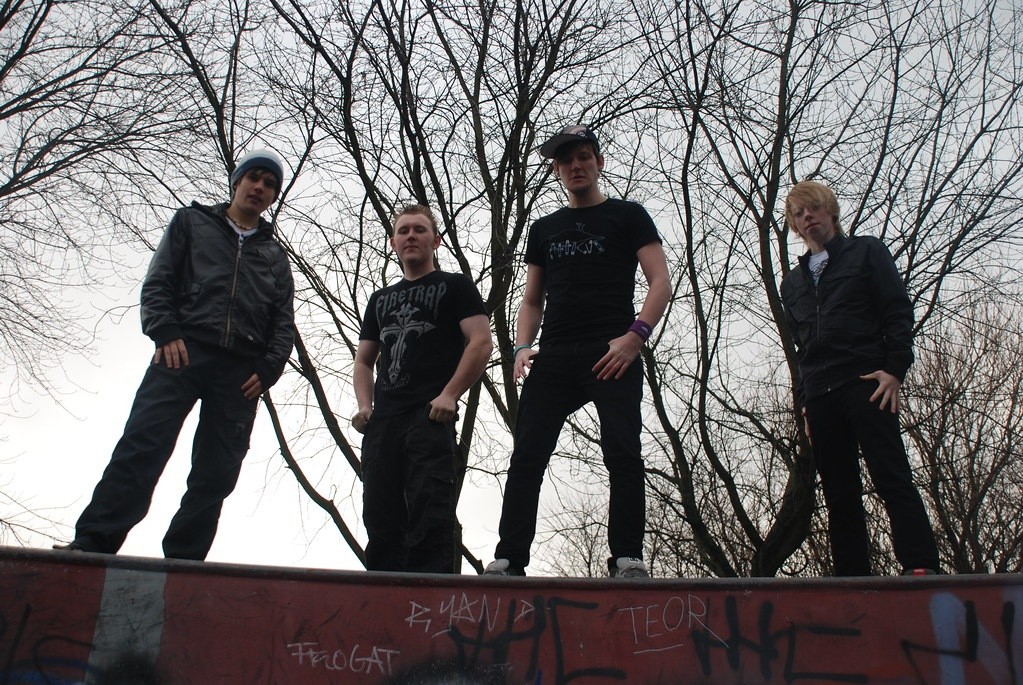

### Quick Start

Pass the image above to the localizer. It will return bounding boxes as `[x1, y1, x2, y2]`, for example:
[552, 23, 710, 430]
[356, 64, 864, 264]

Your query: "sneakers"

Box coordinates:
[484, 558, 525, 577]
[615, 557, 650, 579]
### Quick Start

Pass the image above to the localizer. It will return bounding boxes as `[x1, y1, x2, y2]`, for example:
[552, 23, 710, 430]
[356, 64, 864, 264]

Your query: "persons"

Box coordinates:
[352, 205, 493, 574]
[482, 125, 672, 578]
[52, 150, 295, 562]
[780, 181, 940, 578]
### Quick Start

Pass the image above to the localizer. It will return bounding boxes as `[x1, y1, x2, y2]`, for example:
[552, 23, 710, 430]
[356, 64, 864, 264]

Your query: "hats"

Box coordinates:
[230, 148, 284, 205]
[539, 122, 599, 163]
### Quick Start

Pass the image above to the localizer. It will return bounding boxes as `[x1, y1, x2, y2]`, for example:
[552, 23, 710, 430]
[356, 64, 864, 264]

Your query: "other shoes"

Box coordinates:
[902, 567, 936, 575]
[53, 543, 72, 550]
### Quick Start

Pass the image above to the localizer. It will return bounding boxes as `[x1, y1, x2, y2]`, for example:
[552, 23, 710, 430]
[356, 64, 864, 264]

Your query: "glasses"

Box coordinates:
[576, 130, 600, 153]
